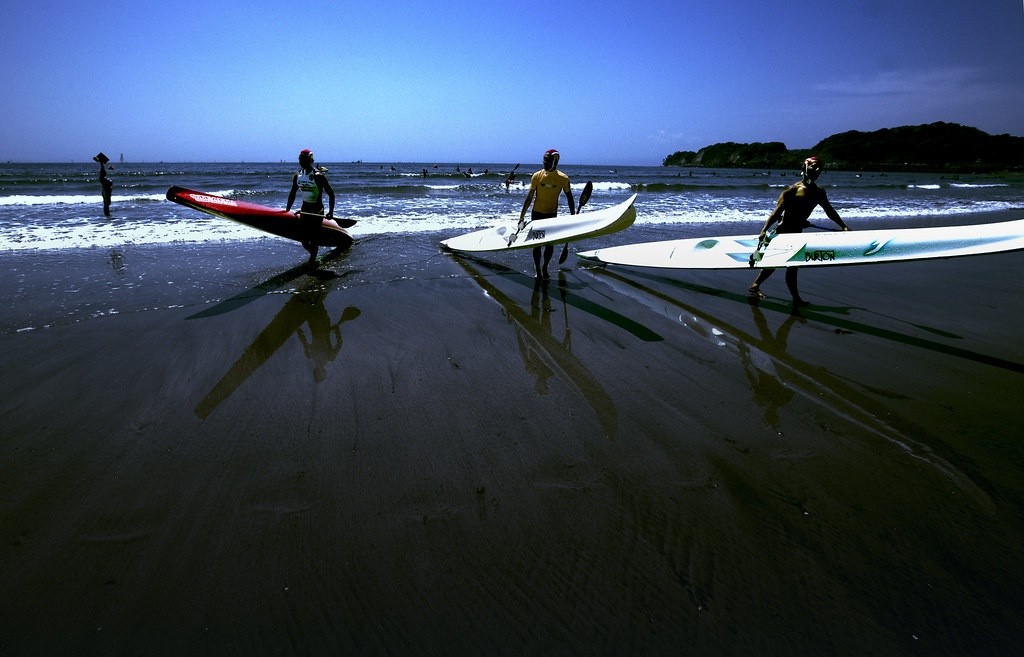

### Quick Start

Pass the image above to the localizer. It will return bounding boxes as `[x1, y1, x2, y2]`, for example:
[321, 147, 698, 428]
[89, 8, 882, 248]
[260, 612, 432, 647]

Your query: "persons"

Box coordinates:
[746, 155, 852, 308]
[285, 148, 335, 276]
[737, 302, 805, 434]
[296, 300, 343, 386]
[505, 279, 570, 390]
[92, 151, 112, 217]
[517, 149, 575, 282]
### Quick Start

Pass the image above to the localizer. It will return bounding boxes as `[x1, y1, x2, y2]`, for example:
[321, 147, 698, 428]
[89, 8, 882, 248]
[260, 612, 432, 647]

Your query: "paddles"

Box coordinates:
[561, 267, 616, 303]
[327, 304, 362, 330]
[777, 215, 842, 232]
[503, 163, 521, 184]
[294, 209, 358, 229]
[799, 289, 964, 340]
[815, 364, 907, 400]
[557, 269, 573, 353]
[557, 180, 593, 264]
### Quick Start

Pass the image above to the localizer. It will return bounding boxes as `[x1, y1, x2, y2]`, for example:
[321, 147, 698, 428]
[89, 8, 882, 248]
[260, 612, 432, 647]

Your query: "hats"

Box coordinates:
[544, 149, 560, 162]
[804, 157, 823, 167]
[300, 149, 314, 160]
